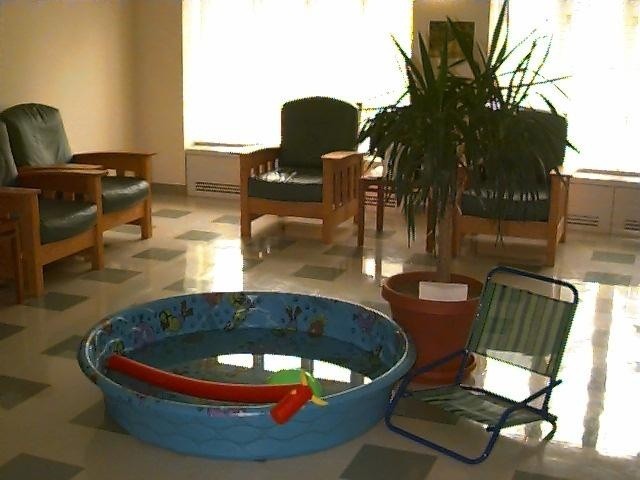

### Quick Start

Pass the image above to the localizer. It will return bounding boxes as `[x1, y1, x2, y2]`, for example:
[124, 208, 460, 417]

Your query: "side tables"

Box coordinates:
[0, 217, 25, 303]
[357, 165, 436, 253]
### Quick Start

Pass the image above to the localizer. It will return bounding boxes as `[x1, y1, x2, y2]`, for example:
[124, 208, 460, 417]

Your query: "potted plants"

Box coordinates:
[352, 0, 580, 386]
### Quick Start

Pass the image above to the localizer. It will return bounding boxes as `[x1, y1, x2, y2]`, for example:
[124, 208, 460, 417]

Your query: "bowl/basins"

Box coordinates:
[77, 291, 418, 461]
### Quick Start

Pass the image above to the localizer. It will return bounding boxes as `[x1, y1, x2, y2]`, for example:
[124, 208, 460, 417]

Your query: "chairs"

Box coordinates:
[453, 106, 573, 267]
[385, 266, 579, 465]
[0, 102, 156, 271]
[239, 96, 367, 244]
[0, 120, 108, 296]
